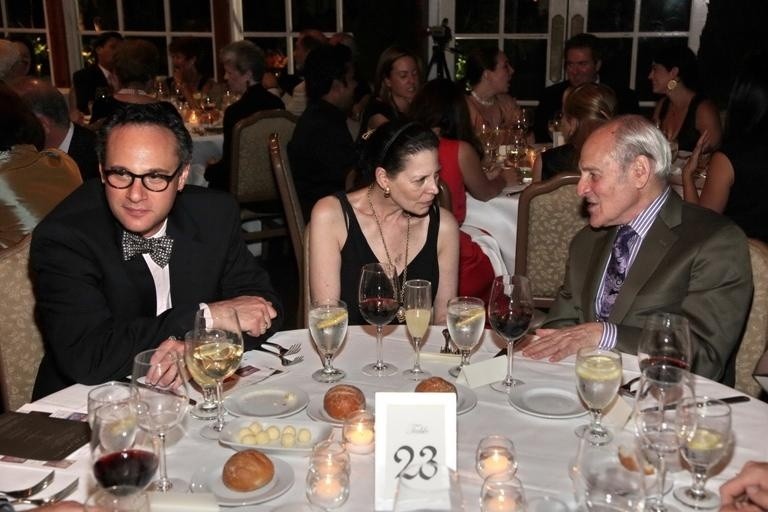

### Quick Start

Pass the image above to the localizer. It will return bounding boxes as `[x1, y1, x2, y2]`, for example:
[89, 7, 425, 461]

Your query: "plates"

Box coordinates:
[221, 384, 311, 419]
[306, 382, 385, 427]
[189, 456, 295, 508]
[400, 379, 477, 416]
[217, 416, 334, 456]
[508, 383, 590, 420]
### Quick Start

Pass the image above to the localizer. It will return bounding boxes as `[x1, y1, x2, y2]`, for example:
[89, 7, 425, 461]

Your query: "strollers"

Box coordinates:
[122, 230, 174, 269]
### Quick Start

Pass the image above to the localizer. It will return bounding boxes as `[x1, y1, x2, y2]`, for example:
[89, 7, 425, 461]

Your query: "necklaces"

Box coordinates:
[368, 182, 410, 324]
[471, 91, 495, 105]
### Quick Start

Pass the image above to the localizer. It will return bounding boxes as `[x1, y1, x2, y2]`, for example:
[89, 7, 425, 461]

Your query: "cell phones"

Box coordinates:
[424, 19, 452, 45]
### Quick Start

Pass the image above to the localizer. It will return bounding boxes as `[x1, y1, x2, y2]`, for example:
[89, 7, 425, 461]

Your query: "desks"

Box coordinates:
[3, 325, 767, 511]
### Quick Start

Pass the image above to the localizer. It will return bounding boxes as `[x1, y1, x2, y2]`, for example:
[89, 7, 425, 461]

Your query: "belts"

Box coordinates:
[201, 96, 217, 130]
[480, 119, 526, 168]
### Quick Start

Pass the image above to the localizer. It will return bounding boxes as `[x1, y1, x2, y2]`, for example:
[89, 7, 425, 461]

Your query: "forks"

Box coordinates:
[0, 469, 80, 506]
[258, 340, 303, 356]
[249, 341, 305, 366]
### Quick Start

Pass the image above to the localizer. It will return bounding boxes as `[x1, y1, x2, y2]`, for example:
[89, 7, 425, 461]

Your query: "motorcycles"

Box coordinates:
[423, 46, 451, 82]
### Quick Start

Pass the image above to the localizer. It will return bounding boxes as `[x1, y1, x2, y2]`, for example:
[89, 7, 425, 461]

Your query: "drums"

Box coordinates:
[641, 396, 750, 412]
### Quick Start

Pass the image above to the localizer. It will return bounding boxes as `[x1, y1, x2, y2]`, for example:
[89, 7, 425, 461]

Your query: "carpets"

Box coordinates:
[598, 225, 639, 323]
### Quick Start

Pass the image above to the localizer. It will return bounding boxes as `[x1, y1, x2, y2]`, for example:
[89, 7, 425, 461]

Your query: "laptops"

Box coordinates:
[416, 377, 455, 392]
[324, 385, 366, 420]
[223, 451, 274, 491]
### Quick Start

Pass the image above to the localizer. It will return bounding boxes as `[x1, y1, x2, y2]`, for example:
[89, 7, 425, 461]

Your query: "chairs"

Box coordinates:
[0, 58, 767, 400]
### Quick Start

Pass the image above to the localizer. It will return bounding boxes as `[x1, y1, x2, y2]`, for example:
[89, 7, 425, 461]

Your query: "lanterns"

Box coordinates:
[582, 355, 617, 374]
[686, 428, 718, 450]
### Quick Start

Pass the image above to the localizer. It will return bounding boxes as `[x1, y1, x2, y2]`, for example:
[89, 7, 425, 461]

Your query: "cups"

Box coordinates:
[670, 142, 679, 163]
[100, 155, 183, 193]
[480, 471, 528, 509]
[342, 410, 380, 455]
[306, 457, 351, 509]
[309, 439, 351, 475]
[513, 108, 535, 137]
[476, 435, 518, 484]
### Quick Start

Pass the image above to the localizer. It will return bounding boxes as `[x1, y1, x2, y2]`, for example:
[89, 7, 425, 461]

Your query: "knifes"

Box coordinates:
[641, 396, 750, 412]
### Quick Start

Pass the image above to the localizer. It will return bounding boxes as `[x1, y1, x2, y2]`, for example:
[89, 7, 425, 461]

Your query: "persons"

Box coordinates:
[532, 82, 617, 184]
[310, 118, 459, 325]
[0, 39, 31, 86]
[286, 44, 364, 222]
[263, 30, 327, 116]
[30, 102, 283, 396]
[522, 114, 752, 389]
[204, 40, 285, 190]
[681, 49, 768, 243]
[73, 31, 125, 114]
[355, 44, 420, 149]
[8, 75, 100, 184]
[409, 78, 523, 326]
[0, 81, 84, 252]
[89, 39, 180, 130]
[719, 460, 768, 512]
[162, 37, 211, 105]
[646, 41, 724, 153]
[463, 46, 526, 144]
[533, 34, 639, 144]
[16, 501, 85, 512]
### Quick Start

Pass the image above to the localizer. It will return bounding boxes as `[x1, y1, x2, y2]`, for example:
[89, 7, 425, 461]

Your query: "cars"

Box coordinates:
[670, 142, 679, 163]
[513, 108, 535, 137]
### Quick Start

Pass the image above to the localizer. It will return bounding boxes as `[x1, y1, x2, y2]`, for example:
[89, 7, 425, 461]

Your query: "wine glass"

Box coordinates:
[631, 364, 700, 512]
[185, 329, 229, 421]
[192, 305, 235, 441]
[570, 425, 647, 509]
[89, 382, 141, 433]
[445, 296, 487, 377]
[304, 298, 349, 383]
[575, 345, 624, 446]
[401, 280, 433, 382]
[88, 397, 163, 494]
[131, 347, 191, 500]
[357, 262, 401, 377]
[89, 485, 153, 509]
[488, 274, 536, 396]
[201, 96, 217, 130]
[480, 119, 526, 168]
[679, 392, 731, 509]
[630, 308, 693, 409]
